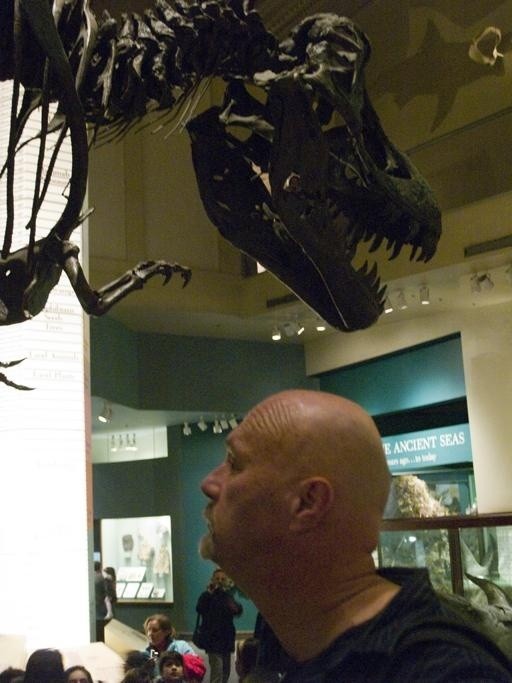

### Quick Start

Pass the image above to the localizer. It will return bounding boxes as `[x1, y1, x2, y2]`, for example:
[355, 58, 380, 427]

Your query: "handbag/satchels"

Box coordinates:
[193, 626, 213, 648]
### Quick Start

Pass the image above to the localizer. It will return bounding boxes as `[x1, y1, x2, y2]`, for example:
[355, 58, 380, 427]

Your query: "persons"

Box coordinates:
[1, 612, 207, 683]
[102, 566, 117, 620]
[196, 390, 511, 682]
[94, 561, 112, 642]
[196, 566, 244, 682]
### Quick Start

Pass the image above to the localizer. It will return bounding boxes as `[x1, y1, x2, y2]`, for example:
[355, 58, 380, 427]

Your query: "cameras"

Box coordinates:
[212, 582, 223, 593]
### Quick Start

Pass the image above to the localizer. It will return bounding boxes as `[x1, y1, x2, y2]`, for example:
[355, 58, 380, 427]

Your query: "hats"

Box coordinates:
[183, 655, 206, 679]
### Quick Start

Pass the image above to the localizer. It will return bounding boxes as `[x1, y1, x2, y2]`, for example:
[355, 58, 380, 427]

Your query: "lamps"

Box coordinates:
[220, 419, 229, 429]
[289, 318, 306, 335]
[316, 316, 326, 332]
[284, 323, 293, 336]
[420, 288, 430, 305]
[213, 420, 222, 433]
[183, 422, 193, 434]
[228, 418, 238, 430]
[397, 293, 407, 310]
[272, 326, 282, 341]
[470, 275, 481, 293]
[467, 27, 504, 66]
[98, 403, 113, 423]
[479, 275, 494, 292]
[198, 417, 208, 432]
[382, 296, 394, 313]
